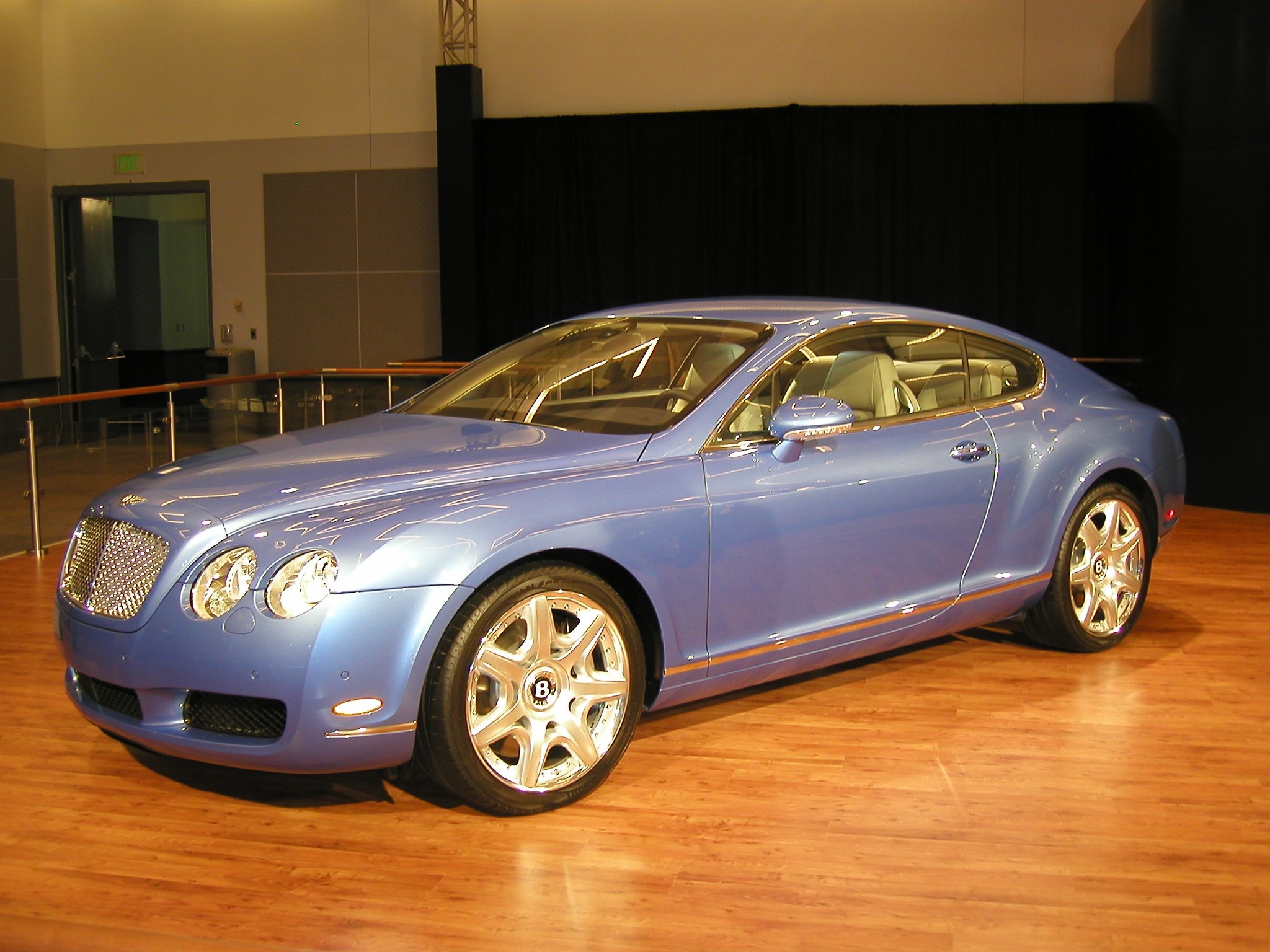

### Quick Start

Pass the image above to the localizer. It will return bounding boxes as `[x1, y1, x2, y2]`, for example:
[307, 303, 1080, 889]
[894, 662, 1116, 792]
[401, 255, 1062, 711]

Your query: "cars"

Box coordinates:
[49, 296, 1184, 815]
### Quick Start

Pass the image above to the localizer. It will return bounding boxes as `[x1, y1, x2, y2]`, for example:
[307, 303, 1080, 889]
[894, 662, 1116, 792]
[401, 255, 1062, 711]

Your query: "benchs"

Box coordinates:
[781, 355, 1004, 414]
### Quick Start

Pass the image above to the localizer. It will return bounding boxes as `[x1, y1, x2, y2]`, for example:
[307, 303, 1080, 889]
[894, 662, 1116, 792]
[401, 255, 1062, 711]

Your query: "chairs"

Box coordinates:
[822, 351, 900, 423]
[674, 344, 764, 433]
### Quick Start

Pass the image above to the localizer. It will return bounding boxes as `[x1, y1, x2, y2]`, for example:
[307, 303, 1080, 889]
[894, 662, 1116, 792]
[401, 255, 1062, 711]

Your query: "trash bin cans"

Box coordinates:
[202, 348, 260, 449]
